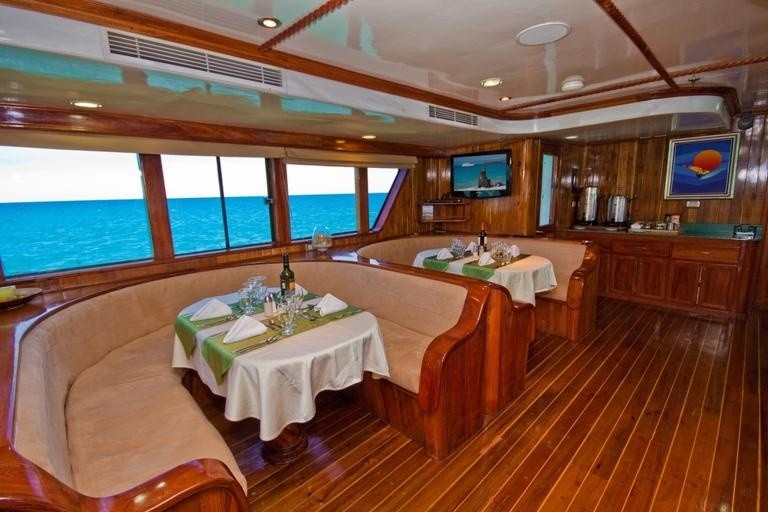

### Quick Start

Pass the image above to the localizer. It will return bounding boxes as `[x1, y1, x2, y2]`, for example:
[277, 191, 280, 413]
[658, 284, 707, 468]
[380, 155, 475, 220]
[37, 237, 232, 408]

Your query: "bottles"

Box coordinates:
[643, 214, 680, 230]
[477, 222, 487, 256]
[279, 254, 295, 305]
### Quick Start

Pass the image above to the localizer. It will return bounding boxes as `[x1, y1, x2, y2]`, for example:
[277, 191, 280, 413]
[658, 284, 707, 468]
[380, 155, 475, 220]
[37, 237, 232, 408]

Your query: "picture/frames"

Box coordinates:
[664, 132, 741, 199]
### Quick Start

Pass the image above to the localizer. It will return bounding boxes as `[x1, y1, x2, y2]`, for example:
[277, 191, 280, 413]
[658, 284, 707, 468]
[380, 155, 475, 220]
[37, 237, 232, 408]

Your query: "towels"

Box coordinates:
[464, 241, 478, 252]
[220, 316, 268, 343]
[478, 252, 495, 266]
[508, 243, 521, 257]
[437, 247, 454, 260]
[189, 296, 233, 321]
[291, 282, 308, 296]
[310, 293, 350, 317]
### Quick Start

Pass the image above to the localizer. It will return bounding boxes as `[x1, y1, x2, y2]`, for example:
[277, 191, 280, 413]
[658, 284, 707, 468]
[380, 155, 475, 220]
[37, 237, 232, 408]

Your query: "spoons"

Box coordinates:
[237, 336, 277, 356]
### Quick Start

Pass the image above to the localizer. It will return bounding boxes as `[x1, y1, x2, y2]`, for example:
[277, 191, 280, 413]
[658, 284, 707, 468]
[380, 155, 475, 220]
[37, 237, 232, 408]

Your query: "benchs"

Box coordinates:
[3, 246, 490, 512]
[332, 230, 601, 414]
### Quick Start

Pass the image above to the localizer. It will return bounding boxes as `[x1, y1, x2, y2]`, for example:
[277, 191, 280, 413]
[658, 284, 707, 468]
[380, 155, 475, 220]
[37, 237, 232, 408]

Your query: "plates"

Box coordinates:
[1, 287, 43, 309]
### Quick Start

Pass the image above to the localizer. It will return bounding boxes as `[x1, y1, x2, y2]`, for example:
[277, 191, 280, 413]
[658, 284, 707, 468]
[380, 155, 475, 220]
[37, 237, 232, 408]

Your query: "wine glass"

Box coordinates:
[237, 276, 303, 336]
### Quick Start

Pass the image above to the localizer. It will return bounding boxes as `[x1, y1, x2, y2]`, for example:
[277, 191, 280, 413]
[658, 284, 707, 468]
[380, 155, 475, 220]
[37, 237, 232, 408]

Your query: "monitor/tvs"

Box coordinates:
[450, 148, 513, 199]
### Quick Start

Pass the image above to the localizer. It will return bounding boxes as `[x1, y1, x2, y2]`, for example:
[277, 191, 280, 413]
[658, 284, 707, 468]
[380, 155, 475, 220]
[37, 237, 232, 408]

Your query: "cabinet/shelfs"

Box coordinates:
[547, 233, 764, 321]
[417, 202, 471, 223]
